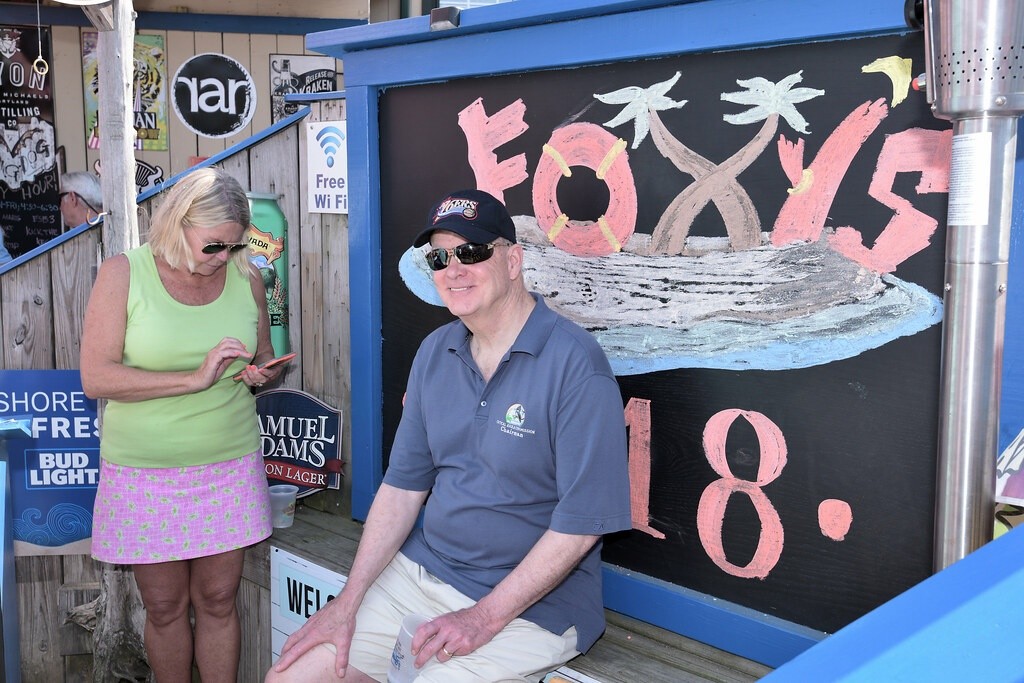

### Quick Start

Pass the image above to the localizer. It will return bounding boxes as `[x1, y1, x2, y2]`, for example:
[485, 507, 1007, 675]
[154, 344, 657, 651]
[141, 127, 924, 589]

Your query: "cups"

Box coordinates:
[267, 484, 299, 528]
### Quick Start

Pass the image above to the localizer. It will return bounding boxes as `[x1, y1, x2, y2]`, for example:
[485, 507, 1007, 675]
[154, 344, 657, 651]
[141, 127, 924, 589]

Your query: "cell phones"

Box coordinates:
[232, 351, 297, 383]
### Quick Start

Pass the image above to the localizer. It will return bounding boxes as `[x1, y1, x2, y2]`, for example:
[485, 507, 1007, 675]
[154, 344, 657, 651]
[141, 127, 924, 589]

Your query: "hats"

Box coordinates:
[413, 188, 518, 248]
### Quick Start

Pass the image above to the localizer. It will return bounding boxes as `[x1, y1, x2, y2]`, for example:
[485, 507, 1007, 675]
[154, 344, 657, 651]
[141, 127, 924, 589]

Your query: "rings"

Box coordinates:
[254, 381, 264, 387]
[441, 646, 453, 657]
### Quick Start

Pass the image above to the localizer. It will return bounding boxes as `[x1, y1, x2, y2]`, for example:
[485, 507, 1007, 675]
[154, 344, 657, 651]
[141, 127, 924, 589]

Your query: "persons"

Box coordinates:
[79, 168, 282, 682]
[262, 193, 634, 683]
[58, 170, 104, 227]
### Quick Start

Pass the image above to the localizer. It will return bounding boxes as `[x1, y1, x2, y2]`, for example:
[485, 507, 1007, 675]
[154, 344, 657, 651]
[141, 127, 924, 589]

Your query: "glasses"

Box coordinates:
[55, 192, 83, 207]
[184, 218, 248, 254]
[425, 243, 513, 271]
